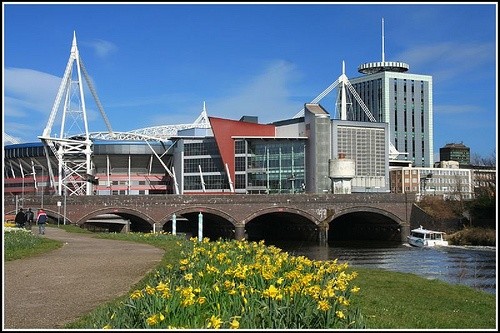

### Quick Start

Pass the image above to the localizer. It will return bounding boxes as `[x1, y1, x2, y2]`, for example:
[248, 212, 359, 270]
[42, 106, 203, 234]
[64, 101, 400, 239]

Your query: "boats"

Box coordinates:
[407, 223, 451, 250]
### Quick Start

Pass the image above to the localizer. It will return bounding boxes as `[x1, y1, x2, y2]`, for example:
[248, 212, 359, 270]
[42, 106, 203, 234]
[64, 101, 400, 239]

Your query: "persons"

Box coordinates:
[23, 207, 34, 235]
[14, 208, 26, 229]
[36, 209, 49, 235]
[299, 182, 307, 194]
